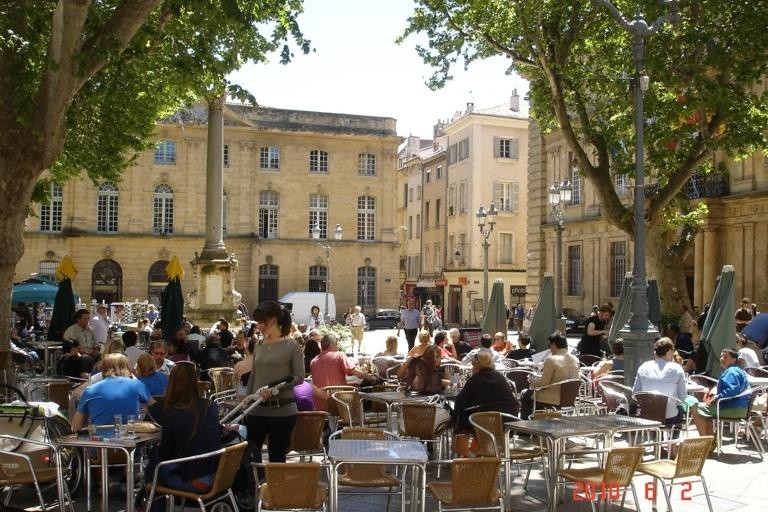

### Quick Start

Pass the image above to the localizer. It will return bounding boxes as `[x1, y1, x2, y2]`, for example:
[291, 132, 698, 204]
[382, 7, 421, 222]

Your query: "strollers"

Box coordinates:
[0, 383, 83, 501]
[210, 373, 292, 511]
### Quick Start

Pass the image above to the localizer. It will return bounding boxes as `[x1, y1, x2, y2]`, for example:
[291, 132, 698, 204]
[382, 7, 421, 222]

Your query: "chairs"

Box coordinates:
[0, 331, 768, 512]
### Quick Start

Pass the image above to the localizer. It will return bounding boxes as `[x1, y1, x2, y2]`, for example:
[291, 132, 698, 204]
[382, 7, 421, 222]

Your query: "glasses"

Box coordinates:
[153, 351, 167, 356]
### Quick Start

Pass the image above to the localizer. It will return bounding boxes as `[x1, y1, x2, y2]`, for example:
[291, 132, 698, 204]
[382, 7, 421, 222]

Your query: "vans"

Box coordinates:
[276, 292, 336, 328]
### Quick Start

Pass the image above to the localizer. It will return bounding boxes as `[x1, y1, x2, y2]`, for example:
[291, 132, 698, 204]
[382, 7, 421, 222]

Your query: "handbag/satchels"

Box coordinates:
[398, 395, 456, 435]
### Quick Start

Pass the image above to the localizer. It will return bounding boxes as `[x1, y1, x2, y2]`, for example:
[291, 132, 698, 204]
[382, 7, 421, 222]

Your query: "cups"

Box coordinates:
[441, 368, 468, 394]
[112, 414, 123, 438]
[86, 417, 96, 438]
[126, 414, 136, 438]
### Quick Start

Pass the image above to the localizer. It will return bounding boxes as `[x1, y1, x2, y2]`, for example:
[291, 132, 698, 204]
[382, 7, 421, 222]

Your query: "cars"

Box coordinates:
[363, 308, 404, 331]
[560, 308, 588, 334]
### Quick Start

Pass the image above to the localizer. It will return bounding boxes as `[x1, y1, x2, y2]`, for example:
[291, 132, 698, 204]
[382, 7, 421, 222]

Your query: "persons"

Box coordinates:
[9, 297, 768, 511]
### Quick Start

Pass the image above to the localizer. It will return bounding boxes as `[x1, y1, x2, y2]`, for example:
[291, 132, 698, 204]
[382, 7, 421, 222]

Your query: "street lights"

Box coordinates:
[310, 221, 342, 319]
[549, 179, 572, 322]
[476, 201, 497, 319]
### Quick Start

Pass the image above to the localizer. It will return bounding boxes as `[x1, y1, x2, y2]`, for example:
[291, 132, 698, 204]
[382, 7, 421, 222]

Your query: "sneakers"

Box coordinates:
[708, 441, 715, 453]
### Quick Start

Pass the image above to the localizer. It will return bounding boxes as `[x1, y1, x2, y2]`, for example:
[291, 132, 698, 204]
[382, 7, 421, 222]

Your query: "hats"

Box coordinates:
[653, 337, 673, 349]
[743, 298, 751, 303]
[474, 353, 479, 360]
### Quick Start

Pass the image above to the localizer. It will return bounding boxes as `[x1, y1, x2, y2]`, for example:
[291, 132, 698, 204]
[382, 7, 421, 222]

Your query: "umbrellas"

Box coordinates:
[527, 273, 557, 346]
[648, 276, 661, 323]
[481, 278, 507, 338]
[48, 252, 79, 341]
[159, 255, 184, 338]
[698, 264, 736, 377]
[610, 272, 632, 342]
[12, 272, 79, 307]
